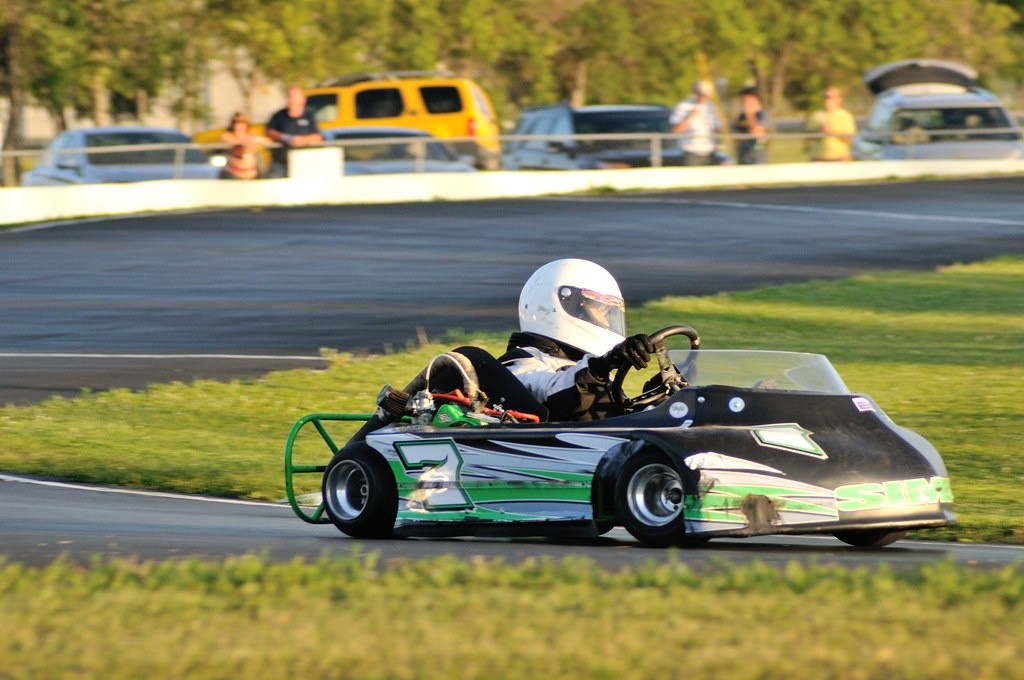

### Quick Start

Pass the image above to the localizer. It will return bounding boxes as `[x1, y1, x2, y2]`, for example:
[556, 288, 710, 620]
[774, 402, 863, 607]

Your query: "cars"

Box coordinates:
[18, 126, 222, 186]
[501, 102, 736, 169]
[847, 59, 1024, 161]
[270, 126, 483, 174]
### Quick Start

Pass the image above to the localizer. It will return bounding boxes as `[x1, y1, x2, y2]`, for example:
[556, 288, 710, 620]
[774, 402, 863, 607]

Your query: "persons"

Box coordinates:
[267, 85, 323, 175]
[219, 113, 271, 180]
[666, 79, 728, 166]
[807, 88, 857, 161]
[731, 88, 770, 163]
[497, 259, 697, 422]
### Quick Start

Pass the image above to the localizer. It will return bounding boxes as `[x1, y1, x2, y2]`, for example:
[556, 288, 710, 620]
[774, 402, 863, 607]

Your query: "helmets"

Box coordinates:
[517, 258, 627, 357]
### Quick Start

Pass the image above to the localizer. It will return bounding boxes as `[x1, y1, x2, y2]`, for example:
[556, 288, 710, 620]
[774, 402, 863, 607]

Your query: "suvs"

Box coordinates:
[192, 70, 505, 174]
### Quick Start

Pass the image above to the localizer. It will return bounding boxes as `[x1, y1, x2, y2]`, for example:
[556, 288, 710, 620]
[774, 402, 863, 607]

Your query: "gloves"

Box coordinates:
[588, 333, 653, 381]
[642, 363, 695, 405]
